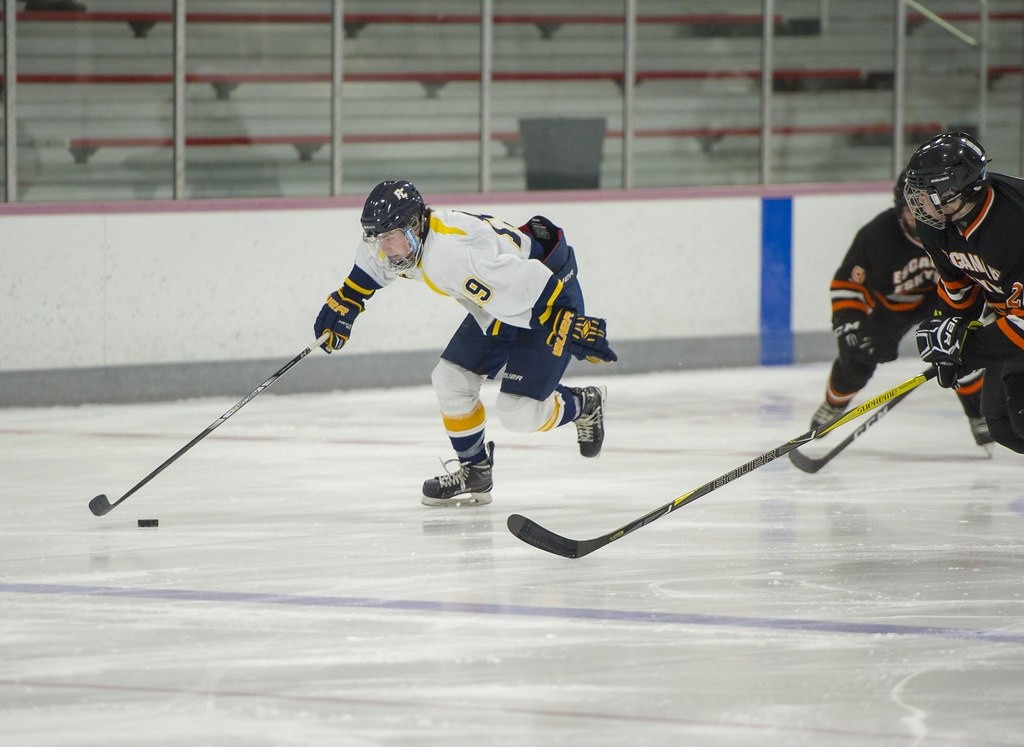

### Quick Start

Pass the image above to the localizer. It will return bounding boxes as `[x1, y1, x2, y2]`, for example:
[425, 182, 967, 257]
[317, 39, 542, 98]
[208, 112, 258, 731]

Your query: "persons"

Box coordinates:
[313, 180, 619, 510]
[901, 128, 1024, 455]
[810, 167, 994, 458]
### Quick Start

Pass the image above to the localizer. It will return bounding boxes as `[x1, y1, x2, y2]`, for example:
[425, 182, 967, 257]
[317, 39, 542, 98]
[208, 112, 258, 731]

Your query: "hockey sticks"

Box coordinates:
[88, 332, 333, 518]
[788, 367, 938, 473]
[505, 368, 940, 560]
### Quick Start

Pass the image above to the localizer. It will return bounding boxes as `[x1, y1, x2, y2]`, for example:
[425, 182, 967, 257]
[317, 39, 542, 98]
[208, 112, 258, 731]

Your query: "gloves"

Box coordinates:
[314, 287, 366, 355]
[568, 316, 618, 364]
[834, 322, 874, 355]
[916, 311, 983, 361]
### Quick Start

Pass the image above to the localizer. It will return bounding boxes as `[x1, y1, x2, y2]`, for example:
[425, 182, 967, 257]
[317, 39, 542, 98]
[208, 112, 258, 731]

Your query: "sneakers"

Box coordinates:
[811, 403, 846, 440]
[423, 441, 495, 498]
[573, 386, 604, 458]
[967, 417, 995, 447]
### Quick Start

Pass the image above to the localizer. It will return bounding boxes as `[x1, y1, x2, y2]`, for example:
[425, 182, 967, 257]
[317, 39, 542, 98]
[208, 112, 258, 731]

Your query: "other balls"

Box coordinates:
[137, 519, 159, 528]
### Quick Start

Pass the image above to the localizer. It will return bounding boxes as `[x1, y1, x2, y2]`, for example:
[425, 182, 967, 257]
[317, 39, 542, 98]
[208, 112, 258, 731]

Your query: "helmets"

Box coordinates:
[360, 180, 424, 234]
[907, 132, 992, 196]
